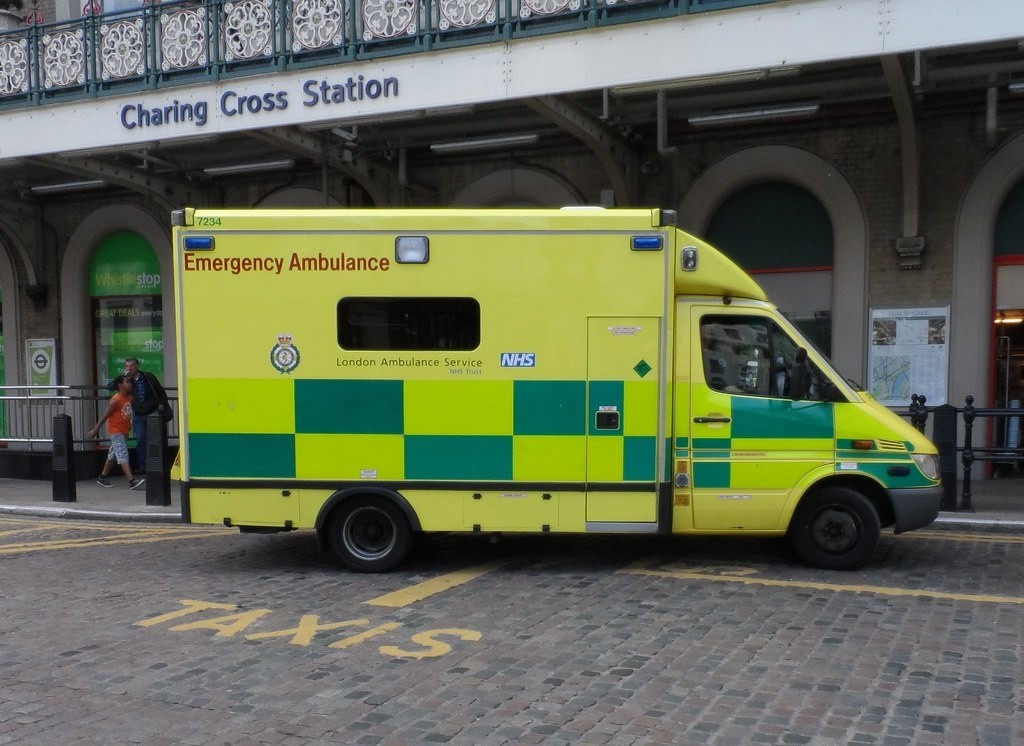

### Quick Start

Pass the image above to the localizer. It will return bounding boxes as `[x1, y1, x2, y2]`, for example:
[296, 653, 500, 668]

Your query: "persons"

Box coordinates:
[123, 357, 174, 476]
[705, 326, 747, 394]
[87, 375, 145, 490]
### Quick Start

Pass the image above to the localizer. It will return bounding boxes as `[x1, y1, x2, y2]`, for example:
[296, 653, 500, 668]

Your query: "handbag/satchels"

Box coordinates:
[157, 403, 173, 422]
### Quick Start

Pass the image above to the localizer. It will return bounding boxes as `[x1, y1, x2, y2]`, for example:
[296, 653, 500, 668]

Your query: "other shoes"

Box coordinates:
[128, 479, 146, 489]
[96, 474, 115, 488]
[134, 467, 146, 474]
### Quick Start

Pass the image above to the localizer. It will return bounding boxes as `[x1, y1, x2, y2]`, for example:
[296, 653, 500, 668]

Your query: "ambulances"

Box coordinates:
[170, 206, 945, 573]
